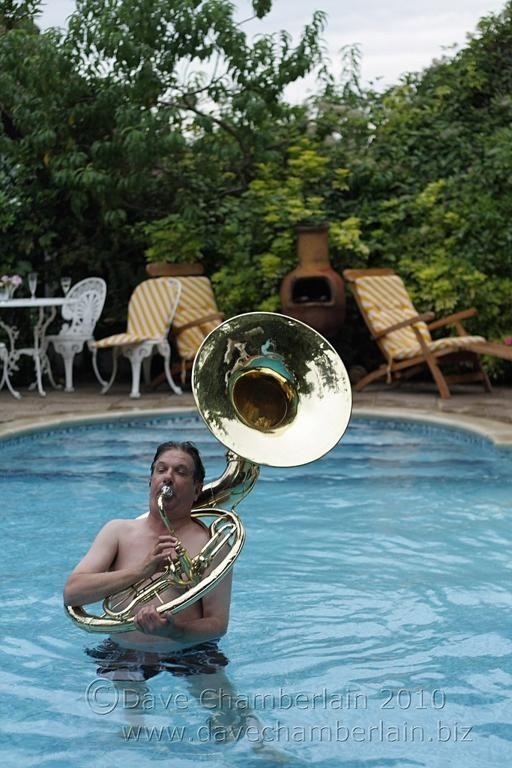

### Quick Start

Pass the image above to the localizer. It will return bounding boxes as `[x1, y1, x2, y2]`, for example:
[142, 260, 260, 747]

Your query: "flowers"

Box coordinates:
[0, 273, 22, 286]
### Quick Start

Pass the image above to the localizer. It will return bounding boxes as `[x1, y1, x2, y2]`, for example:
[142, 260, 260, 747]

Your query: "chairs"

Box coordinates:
[30, 276, 109, 392]
[343, 266, 512, 400]
[90, 276, 184, 396]
[144, 260, 225, 391]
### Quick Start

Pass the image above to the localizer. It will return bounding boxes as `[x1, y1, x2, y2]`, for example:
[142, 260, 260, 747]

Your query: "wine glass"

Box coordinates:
[29, 272, 38, 299]
[61, 277, 72, 298]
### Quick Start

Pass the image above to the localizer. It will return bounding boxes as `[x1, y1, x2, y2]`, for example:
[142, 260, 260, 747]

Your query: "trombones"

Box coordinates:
[63, 310, 354, 634]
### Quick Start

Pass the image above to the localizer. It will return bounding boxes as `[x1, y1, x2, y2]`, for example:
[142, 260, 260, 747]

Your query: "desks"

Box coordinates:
[0, 296, 82, 397]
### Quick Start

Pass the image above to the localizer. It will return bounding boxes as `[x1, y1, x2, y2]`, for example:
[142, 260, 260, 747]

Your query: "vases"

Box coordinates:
[1, 285, 15, 299]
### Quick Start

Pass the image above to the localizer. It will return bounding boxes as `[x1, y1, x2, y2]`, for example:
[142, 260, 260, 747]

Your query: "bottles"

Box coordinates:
[282, 221, 348, 358]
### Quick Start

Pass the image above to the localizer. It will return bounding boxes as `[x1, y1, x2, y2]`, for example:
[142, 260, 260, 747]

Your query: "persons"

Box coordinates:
[62, 439, 234, 681]
[115, 674, 248, 746]
